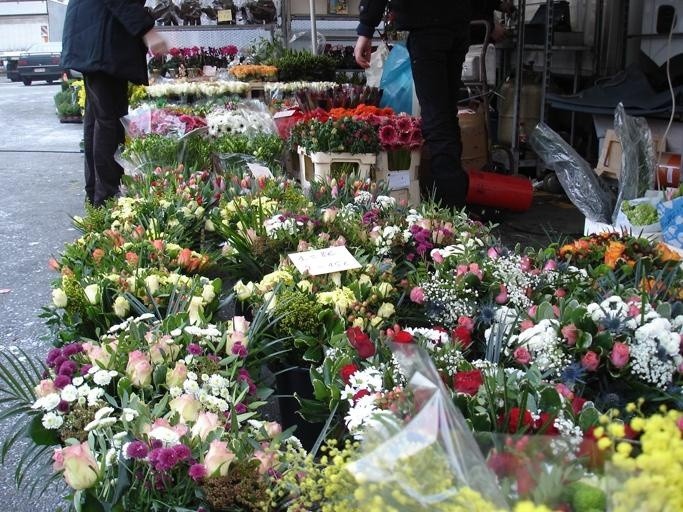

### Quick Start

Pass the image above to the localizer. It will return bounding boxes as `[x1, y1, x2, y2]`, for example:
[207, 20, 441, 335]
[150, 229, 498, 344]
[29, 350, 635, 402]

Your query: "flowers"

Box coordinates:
[0, 162, 683, 511]
[112, 46, 425, 198]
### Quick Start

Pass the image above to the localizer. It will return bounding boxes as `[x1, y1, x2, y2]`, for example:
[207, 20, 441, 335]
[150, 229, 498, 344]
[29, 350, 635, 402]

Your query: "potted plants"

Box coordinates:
[53, 80, 84, 124]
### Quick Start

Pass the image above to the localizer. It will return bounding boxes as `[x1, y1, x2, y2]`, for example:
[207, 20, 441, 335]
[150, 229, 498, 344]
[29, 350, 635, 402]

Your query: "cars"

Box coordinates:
[18, 42, 62, 85]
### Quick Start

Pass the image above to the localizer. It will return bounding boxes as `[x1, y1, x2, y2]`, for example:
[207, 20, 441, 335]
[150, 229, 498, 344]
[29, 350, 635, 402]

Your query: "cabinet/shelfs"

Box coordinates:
[513, 0, 585, 180]
[286, 0, 396, 54]
[144, 0, 288, 59]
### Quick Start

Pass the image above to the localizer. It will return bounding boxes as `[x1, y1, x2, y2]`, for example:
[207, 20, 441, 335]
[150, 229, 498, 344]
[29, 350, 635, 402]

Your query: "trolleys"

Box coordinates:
[456, 21, 514, 176]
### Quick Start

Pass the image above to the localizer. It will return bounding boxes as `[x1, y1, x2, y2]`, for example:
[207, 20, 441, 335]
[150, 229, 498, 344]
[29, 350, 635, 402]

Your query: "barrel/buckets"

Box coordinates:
[271, 361, 332, 458]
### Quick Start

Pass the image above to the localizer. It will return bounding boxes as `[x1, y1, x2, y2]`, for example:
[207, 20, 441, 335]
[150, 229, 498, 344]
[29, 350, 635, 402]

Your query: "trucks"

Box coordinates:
[0, 0, 68, 82]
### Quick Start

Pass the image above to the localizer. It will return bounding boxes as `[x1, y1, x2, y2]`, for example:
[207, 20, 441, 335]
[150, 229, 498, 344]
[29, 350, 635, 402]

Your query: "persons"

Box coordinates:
[58, 0, 169, 212]
[352, 0, 515, 209]
